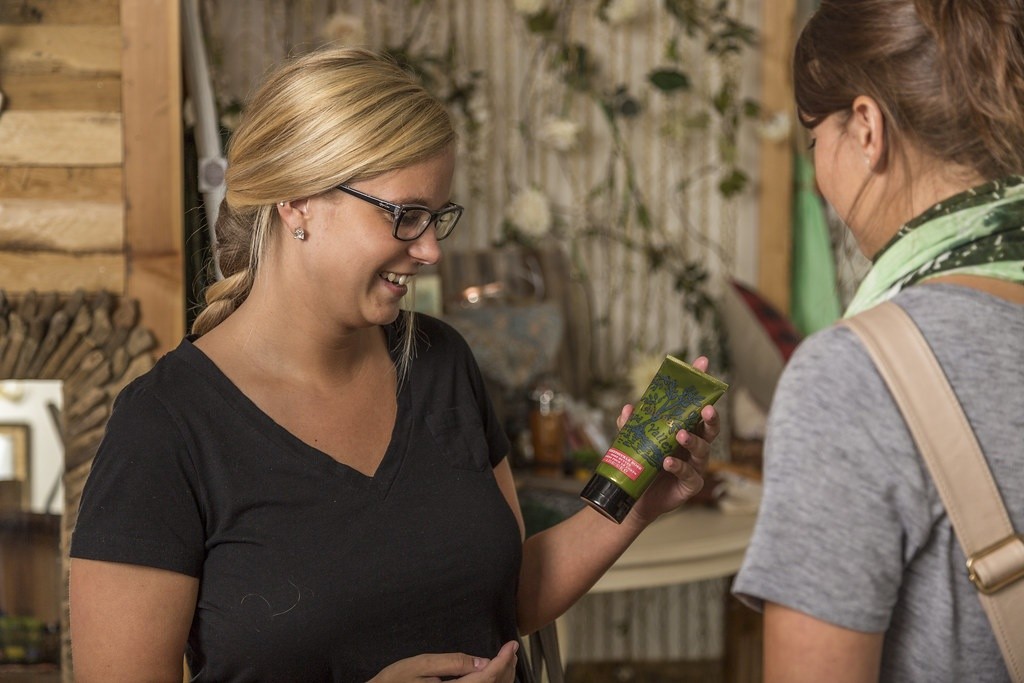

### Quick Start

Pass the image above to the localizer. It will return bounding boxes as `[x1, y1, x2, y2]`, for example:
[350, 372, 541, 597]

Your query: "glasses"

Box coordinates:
[336, 184, 463, 240]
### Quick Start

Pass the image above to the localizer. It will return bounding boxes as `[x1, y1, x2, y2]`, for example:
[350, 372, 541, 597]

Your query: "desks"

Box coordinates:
[556, 504, 754, 665]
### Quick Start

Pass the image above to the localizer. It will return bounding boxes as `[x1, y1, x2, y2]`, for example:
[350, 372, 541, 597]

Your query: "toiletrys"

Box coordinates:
[578, 354, 731, 526]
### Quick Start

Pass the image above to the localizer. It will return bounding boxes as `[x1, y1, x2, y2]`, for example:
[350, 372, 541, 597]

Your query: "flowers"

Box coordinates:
[328, 0, 797, 242]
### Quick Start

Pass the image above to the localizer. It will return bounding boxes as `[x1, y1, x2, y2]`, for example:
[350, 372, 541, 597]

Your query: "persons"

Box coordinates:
[730, 0, 1024, 683]
[67, 45, 719, 683]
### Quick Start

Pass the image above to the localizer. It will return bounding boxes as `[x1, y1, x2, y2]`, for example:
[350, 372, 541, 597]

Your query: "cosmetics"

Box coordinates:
[577, 355, 728, 524]
